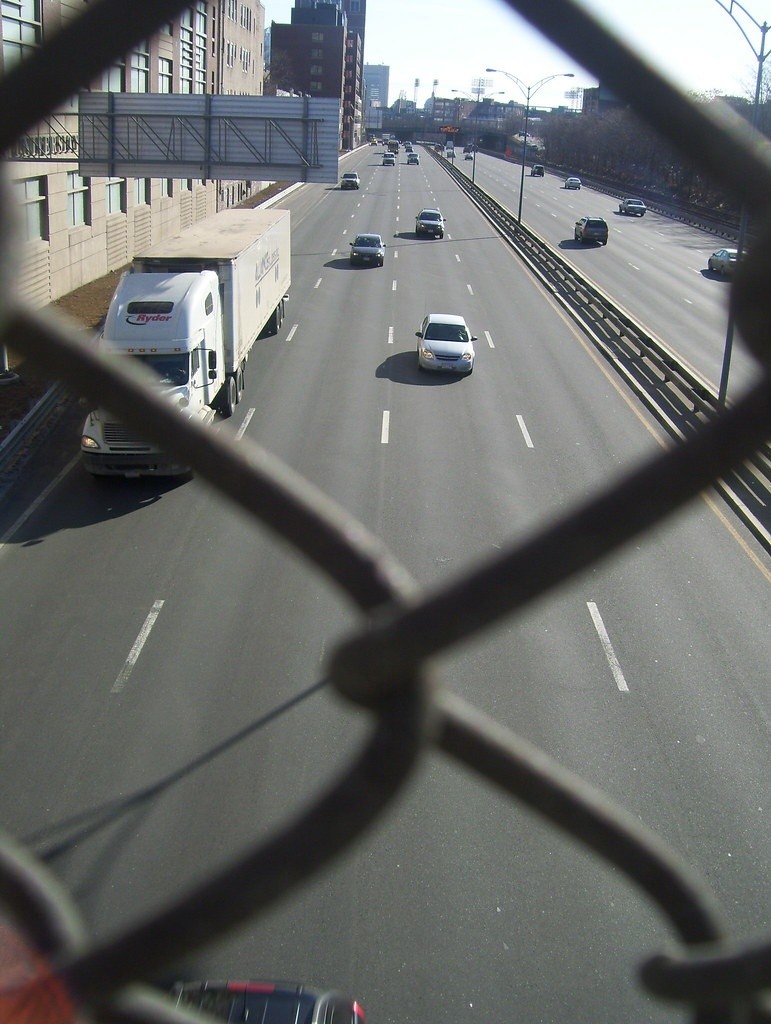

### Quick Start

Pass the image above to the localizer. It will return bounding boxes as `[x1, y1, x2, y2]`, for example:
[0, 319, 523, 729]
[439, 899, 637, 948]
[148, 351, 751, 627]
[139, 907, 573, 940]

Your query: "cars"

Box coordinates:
[619, 199, 648, 217]
[341, 172, 361, 190]
[382, 153, 397, 166]
[168, 979, 365, 1024]
[415, 313, 478, 375]
[406, 153, 421, 166]
[349, 234, 387, 266]
[464, 152, 474, 160]
[435, 140, 479, 157]
[565, 177, 582, 191]
[708, 248, 746, 279]
[370, 134, 413, 152]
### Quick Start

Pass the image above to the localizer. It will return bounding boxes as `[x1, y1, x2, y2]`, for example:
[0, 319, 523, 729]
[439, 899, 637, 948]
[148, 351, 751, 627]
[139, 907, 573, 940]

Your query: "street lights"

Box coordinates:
[452, 89, 505, 181]
[487, 68, 575, 225]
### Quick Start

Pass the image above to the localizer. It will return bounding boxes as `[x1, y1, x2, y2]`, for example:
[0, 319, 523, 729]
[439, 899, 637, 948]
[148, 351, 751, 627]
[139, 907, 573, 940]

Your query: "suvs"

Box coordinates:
[574, 217, 609, 245]
[415, 208, 447, 239]
[531, 165, 545, 177]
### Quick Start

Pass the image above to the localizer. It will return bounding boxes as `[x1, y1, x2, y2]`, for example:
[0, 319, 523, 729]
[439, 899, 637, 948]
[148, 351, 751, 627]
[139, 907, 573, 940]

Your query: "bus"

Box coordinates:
[388, 140, 401, 154]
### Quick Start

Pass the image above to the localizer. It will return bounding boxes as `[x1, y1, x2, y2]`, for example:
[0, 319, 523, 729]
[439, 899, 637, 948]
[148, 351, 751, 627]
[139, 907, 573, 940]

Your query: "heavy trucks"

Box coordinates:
[77, 208, 291, 483]
